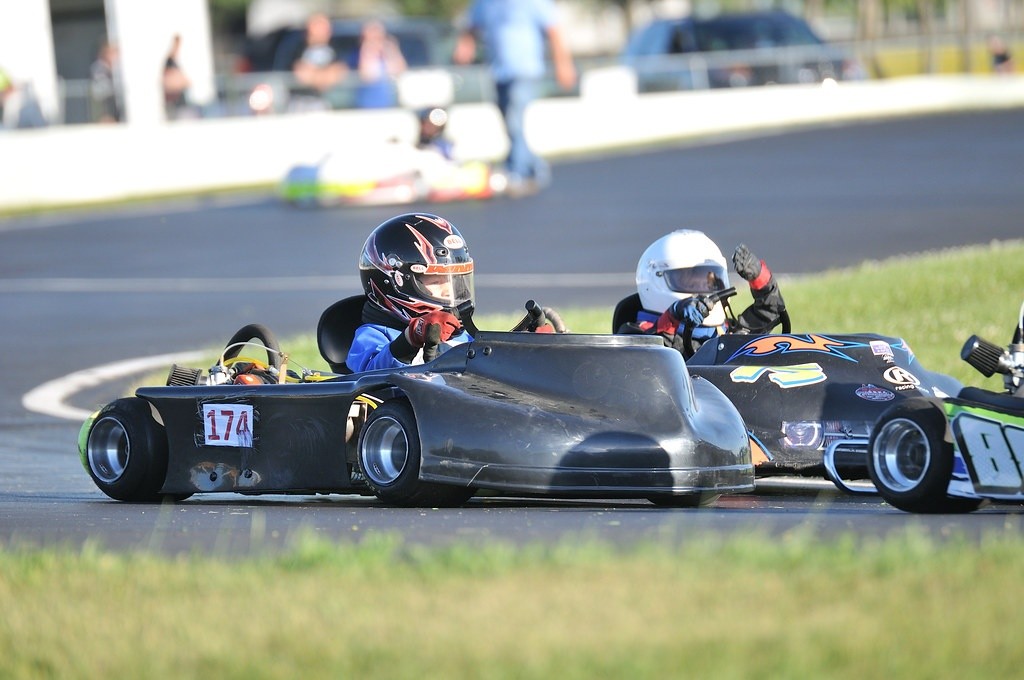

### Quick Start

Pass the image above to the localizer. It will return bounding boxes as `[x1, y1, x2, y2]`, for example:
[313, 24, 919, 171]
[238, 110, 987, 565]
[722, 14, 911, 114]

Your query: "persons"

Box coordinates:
[0, 0, 453, 155]
[990, 37, 1013, 73]
[616, 230, 787, 363]
[345, 212, 554, 373]
[456, 0, 575, 199]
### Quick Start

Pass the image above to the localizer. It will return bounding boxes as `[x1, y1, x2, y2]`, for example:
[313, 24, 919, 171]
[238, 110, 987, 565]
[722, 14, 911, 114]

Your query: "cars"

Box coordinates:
[621, 9, 866, 93]
[212, 0, 581, 109]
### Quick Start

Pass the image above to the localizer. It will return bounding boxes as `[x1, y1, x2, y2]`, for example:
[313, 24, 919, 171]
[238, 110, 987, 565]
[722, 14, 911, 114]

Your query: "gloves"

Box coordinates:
[535, 323, 553, 333]
[732, 244, 762, 280]
[669, 294, 714, 328]
[408, 310, 460, 342]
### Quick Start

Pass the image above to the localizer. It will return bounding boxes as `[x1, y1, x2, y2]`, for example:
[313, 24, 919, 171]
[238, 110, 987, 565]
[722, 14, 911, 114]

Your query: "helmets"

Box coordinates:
[359, 212, 476, 326]
[636, 229, 731, 326]
